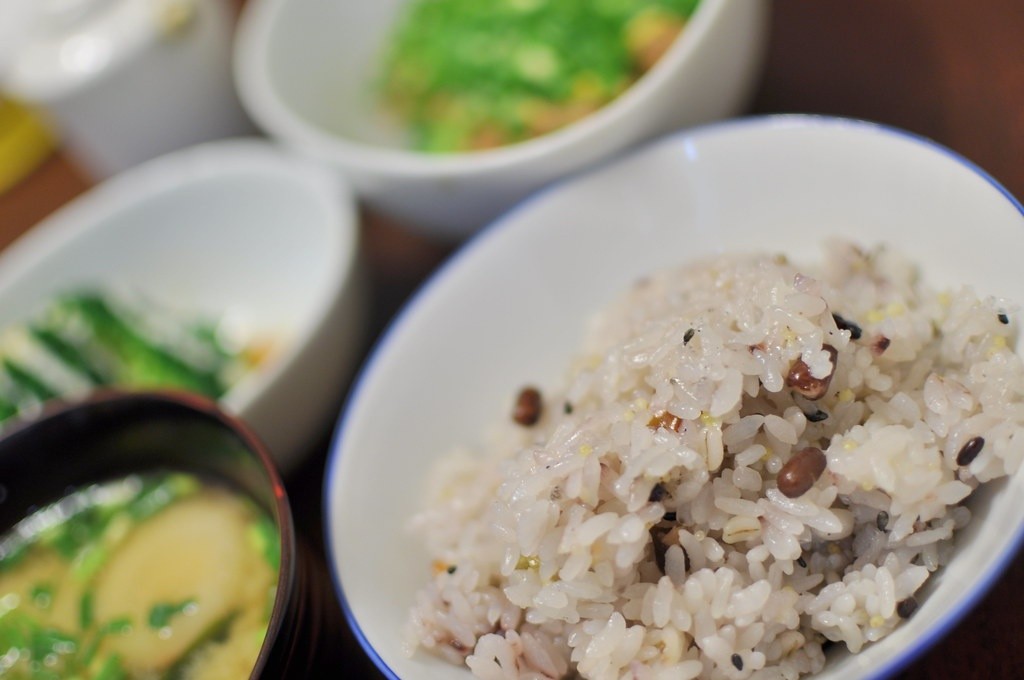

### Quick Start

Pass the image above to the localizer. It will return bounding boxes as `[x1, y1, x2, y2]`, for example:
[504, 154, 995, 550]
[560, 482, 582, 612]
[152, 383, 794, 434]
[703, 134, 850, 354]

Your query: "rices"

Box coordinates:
[409, 242, 1024, 680]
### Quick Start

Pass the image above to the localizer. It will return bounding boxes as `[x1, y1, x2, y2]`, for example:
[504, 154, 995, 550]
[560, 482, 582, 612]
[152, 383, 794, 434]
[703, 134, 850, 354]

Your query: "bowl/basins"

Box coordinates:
[0, 386, 316, 680]
[0, 139, 369, 474]
[230, 0, 769, 236]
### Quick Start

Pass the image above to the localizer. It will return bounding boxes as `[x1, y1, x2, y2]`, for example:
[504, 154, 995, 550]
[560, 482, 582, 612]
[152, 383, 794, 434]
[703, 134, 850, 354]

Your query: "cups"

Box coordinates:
[0, 0, 267, 188]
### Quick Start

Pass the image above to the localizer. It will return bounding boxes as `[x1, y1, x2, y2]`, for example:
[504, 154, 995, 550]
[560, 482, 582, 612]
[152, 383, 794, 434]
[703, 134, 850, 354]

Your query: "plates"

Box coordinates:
[325, 114, 1024, 680]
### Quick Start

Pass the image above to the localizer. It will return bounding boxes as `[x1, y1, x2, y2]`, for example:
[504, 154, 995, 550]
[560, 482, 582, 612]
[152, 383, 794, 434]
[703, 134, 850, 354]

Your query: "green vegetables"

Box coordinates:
[376, 0, 704, 148]
[0, 291, 283, 680]
